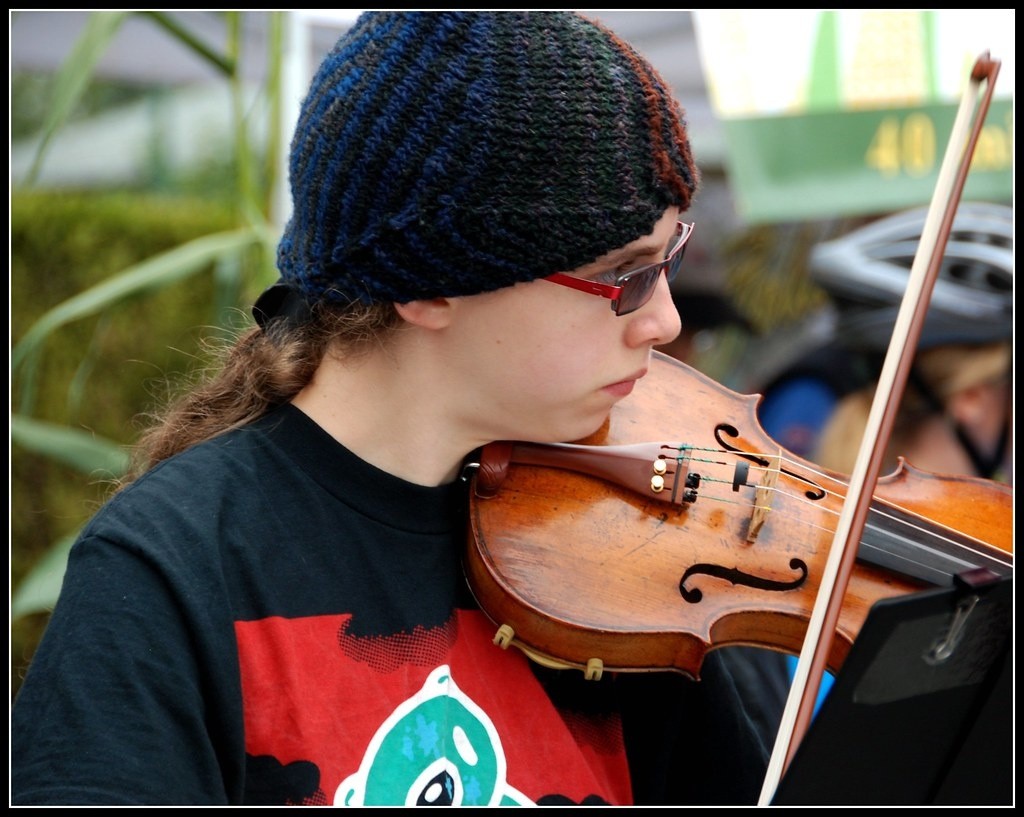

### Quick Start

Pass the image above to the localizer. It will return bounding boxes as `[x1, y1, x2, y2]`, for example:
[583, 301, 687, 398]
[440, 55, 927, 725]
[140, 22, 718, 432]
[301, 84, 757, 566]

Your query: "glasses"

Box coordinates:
[545, 222, 695, 316]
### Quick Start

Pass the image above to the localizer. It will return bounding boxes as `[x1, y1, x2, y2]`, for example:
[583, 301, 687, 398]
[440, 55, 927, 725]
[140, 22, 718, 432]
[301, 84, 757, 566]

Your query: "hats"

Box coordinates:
[277, 11, 698, 309]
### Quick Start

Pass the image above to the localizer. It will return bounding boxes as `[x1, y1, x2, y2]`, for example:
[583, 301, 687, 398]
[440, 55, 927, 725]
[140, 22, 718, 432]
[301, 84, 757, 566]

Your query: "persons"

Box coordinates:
[11, 1, 1013, 807]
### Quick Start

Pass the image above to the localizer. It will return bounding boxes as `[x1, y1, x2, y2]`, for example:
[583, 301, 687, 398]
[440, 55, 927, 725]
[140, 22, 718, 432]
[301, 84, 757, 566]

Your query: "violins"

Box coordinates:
[460, 346, 1015, 683]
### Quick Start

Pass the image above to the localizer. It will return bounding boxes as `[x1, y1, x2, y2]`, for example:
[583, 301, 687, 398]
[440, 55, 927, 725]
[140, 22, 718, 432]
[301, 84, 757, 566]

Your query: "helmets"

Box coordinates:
[808, 201, 1014, 349]
